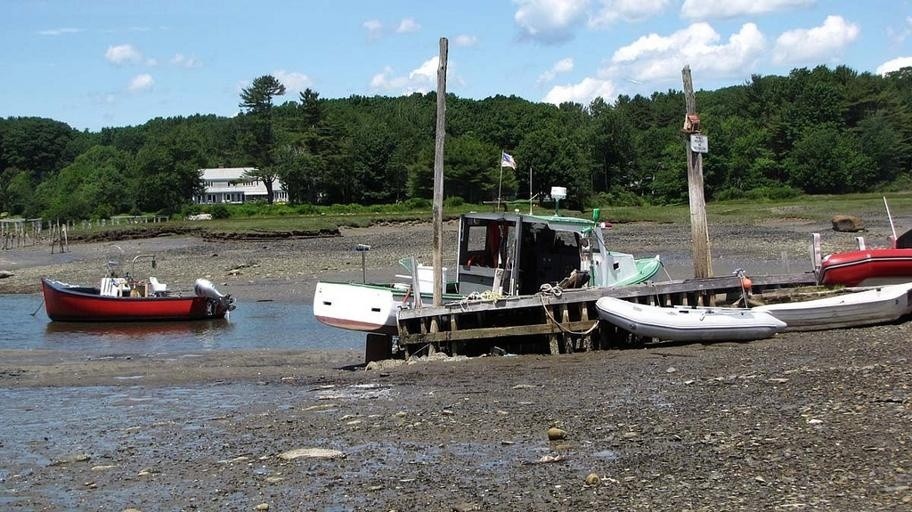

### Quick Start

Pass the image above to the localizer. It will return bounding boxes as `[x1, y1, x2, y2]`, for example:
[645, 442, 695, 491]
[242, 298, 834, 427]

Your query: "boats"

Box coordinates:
[39, 277, 234, 322]
[313, 158, 664, 342]
[753, 280, 912, 331]
[815, 230, 910, 292]
[594, 294, 787, 346]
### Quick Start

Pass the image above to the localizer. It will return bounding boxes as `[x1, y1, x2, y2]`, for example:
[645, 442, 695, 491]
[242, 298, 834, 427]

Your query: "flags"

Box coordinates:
[502, 153, 516, 170]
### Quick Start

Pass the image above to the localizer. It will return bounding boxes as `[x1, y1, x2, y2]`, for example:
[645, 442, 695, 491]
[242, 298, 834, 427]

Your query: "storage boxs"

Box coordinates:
[416, 280, 447, 294]
[416, 266, 447, 283]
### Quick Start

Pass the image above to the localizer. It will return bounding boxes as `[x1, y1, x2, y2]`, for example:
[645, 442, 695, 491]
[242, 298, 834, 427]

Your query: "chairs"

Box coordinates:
[149, 277, 167, 297]
